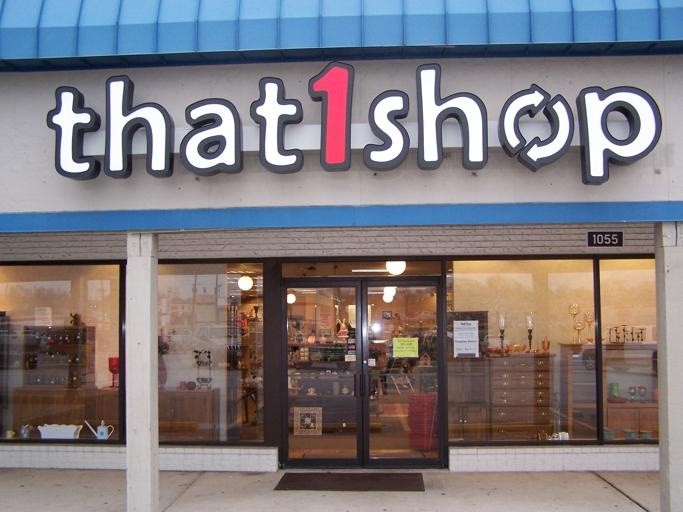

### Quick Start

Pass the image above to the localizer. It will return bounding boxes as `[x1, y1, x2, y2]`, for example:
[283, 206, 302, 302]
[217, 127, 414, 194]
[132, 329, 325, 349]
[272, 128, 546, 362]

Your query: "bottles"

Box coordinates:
[611, 382, 620, 398]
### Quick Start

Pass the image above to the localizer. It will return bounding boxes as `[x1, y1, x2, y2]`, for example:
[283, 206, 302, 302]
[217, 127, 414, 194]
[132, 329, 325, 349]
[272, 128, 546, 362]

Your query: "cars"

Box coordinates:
[576, 324, 657, 373]
[29, 359, 110, 400]
[160, 323, 264, 369]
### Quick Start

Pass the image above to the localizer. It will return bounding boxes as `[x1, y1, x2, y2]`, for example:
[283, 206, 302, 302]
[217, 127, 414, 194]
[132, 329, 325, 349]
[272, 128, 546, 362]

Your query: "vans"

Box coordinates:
[36, 325, 98, 357]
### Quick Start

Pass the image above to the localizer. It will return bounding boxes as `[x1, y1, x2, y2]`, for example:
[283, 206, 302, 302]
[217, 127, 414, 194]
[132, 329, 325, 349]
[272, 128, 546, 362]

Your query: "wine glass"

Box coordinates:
[109, 357, 119, 389]
[628, 386, 647, 403]
[569, 303, 593, 345]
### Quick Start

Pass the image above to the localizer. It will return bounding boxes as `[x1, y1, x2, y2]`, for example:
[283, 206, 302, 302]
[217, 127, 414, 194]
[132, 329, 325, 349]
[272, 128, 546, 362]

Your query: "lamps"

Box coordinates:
[525, 312, 539, 353]
[496, 312, 509, 353]
[566, 301, 595, 346]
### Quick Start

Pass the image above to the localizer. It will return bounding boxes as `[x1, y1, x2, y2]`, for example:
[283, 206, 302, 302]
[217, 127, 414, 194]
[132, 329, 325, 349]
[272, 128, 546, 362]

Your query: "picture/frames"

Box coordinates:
[293, 406, 323, 437]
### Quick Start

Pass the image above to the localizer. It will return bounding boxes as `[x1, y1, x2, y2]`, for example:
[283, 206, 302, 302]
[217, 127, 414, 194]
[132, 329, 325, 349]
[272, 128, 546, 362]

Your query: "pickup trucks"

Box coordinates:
[0, 322, 40, 372]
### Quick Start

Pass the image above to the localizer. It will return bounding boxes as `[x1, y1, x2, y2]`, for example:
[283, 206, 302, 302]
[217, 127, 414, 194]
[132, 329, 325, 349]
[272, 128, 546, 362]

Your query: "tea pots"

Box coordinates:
[84, 420, 115, 439]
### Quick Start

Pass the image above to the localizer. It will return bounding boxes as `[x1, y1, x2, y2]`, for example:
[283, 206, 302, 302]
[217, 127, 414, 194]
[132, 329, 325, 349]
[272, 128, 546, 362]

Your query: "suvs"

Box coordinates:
[290, 362, 411, 439]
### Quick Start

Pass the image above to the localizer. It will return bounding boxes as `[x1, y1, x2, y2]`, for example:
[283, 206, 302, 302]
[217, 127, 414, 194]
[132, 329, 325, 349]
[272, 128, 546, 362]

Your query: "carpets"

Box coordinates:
[273, 473, 425, 491]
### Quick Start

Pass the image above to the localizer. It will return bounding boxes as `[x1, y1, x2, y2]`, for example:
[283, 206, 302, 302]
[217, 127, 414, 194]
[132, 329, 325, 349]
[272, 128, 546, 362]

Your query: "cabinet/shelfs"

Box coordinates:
[288, 340, 385, 432]
[558, 340, 659, 440]
[448, 349, 556, 441]
[21, 325, 96, 388]
[11, 388, 221, 441]
